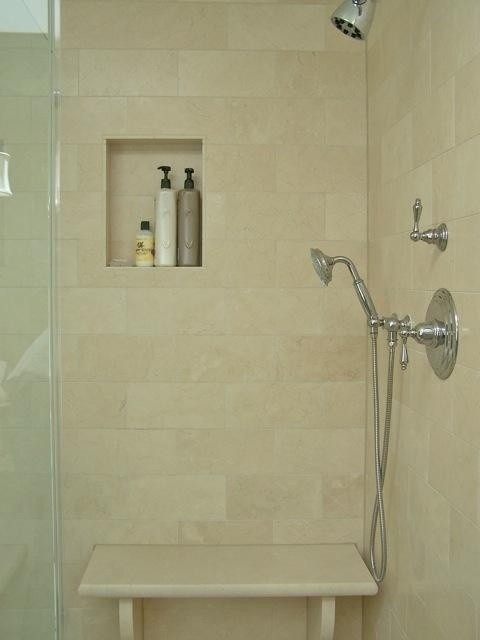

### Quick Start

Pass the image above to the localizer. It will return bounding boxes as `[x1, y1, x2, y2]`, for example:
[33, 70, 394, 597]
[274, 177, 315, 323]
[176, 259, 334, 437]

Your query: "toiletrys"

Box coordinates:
[153, 166, 177, 267]
[177, 168, 202, 267]
